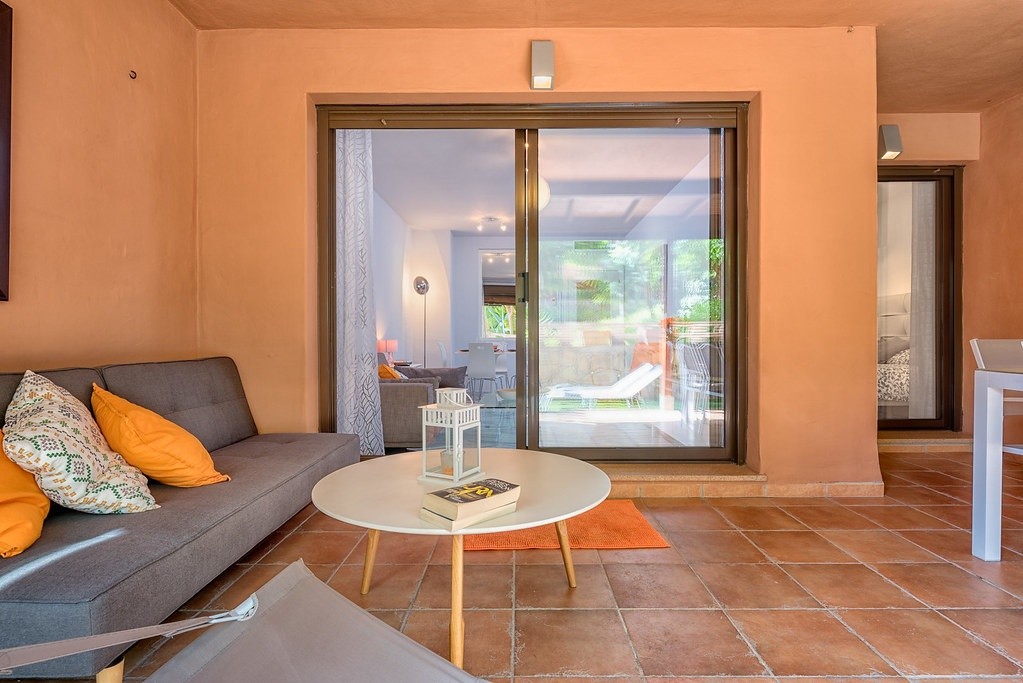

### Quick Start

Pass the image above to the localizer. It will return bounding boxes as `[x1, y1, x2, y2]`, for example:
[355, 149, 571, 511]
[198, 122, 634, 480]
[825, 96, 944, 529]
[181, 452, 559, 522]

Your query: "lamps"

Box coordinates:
[415, 385, 485, 502]
[878, 124, 902, 161]
[377, 340, 398, 367]
[476, 216, 507, 232]
[487, 251, 510, 263]
[529, 39, 555, 91]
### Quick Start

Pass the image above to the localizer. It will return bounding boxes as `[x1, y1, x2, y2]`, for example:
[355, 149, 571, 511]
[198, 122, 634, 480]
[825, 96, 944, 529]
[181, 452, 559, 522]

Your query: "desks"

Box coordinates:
[454, 350, 516, 396]
[972, 369, 1023, 563]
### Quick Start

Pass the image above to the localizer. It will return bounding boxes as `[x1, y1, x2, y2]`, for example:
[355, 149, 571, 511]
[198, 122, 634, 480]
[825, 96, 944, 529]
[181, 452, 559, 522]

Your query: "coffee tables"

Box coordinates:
[311, 446, 612, 675]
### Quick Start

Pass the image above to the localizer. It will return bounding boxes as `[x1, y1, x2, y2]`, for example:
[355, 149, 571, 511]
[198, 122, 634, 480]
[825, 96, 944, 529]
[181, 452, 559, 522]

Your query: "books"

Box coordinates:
[418, 478, 520, 533]
[394, 361, 412, 366]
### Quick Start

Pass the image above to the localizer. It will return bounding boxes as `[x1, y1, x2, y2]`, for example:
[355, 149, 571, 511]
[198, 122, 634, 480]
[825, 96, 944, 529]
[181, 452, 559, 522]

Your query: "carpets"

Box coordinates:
[463, 498, 672, 550]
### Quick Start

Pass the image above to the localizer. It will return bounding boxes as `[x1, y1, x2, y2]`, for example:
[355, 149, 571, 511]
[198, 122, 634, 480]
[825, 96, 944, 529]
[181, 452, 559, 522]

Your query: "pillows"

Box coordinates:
[0, 428, 50, 560]
[392, 368, 409, 379]
[2, 369, 161, 516]
[877, 335, 910, 363]
[378, 363, 400, 379]
[90, 381, 232, 490]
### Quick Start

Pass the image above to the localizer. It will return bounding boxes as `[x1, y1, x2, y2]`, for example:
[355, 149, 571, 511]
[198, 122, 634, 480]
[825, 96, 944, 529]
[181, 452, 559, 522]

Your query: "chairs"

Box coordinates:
[676, 342, 726, 437]
[970, 337, 1023, 417]
[0, 526, 494, 683]
[495, 342, 510, 390]
[467, 342, 498, 402]
[539, 363, 663, 412]
[436, 342, 472, 394]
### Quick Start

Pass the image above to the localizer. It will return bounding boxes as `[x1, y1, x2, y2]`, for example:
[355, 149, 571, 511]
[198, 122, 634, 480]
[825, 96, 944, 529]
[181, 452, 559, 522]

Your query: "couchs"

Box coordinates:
[0, 354, 362, 683]
[877, 292, 910, 420]
[377, 352, 468, 451]
